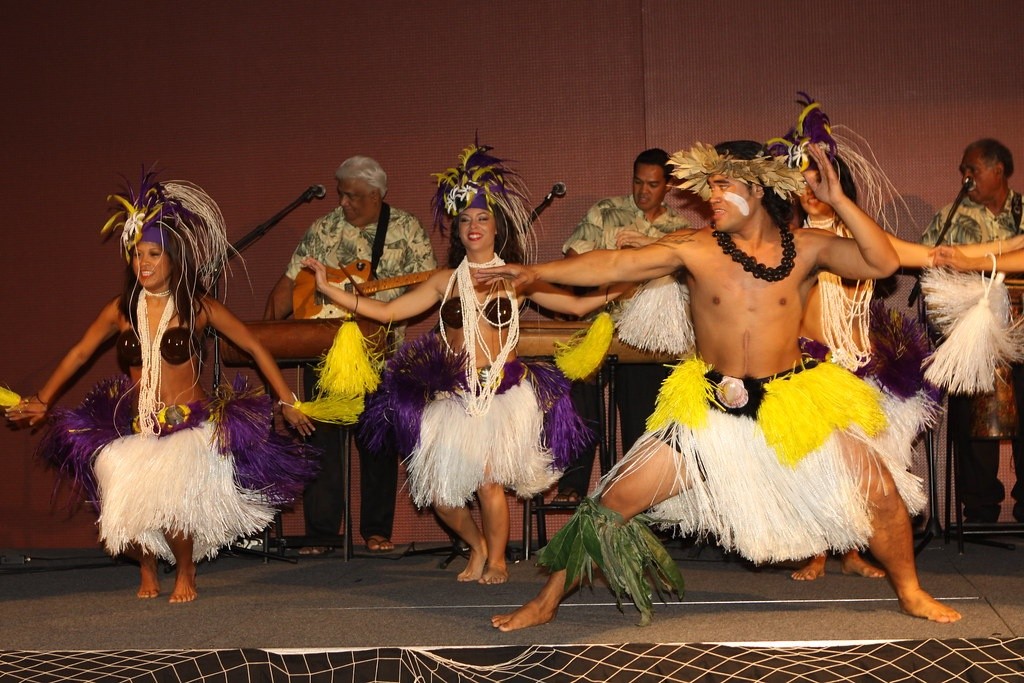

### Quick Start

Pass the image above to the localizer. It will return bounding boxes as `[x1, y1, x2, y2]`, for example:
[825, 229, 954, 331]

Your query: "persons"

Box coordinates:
[552, 148, 689, 505]
[478, 141, 962, 630]
[266, 157, 440, 552]
[300, 145, 636, 582]
[923, 139, 1024, 534]
[615, 91, 1024, 581]
[929, 244, 1024, 274]
[6, 161, 315, 602]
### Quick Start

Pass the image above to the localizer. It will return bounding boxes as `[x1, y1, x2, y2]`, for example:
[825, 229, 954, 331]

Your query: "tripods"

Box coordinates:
[909, 185, 1015, 562]
[404, 532, 468, 569]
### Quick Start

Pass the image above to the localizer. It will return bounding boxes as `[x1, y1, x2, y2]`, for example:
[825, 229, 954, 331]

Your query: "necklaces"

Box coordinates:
[137, 285, 173, 438]
[802, 216, 872, 373]
[441, 252, 520, 403]
[711, 217, 797, 282]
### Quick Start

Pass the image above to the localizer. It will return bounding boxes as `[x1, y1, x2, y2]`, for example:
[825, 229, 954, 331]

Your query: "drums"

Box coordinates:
[961, 274, 1024, 442]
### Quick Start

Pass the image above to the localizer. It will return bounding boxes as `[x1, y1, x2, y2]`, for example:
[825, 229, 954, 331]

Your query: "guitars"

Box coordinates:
[292, 258, 445, 322]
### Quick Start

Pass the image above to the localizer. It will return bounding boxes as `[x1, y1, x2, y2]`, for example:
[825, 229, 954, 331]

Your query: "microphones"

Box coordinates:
[965, 176, 976, 191]
[311, 184, 326, 197]
[553, 181, 567, 195]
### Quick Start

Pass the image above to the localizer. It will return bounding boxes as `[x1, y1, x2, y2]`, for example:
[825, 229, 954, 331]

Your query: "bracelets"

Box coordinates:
[36, 391, 50, 405]
[353, 293, 359, 316]
